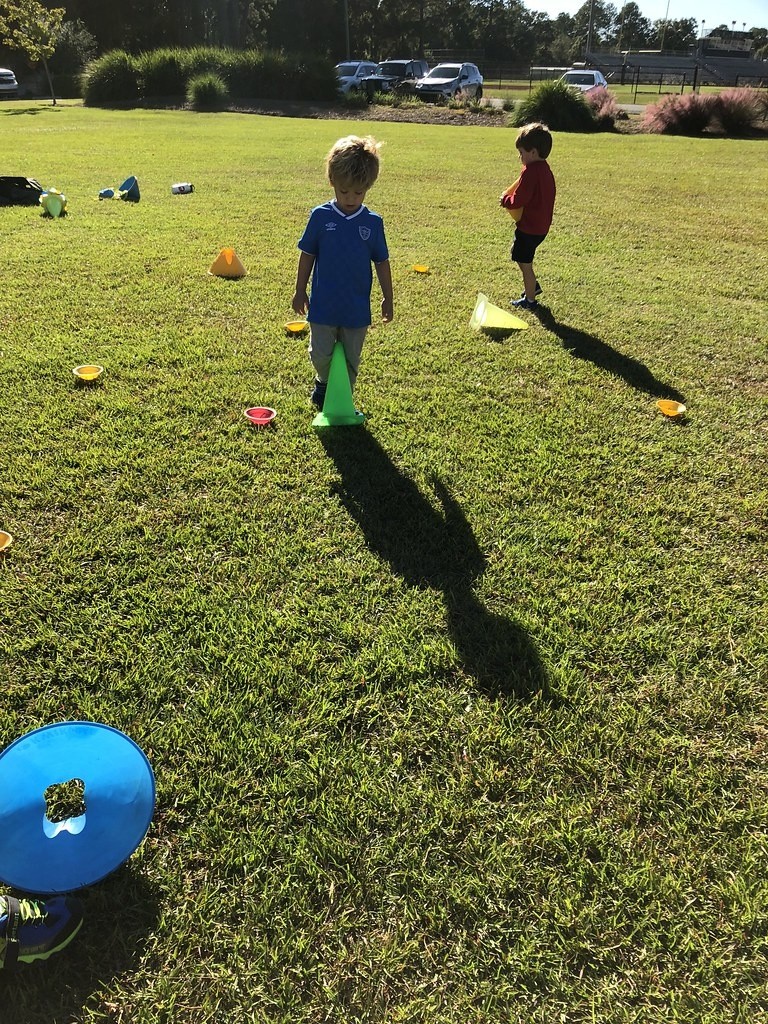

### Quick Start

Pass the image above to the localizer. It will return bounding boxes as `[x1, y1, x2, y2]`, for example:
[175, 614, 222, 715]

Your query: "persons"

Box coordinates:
[292, 135, 393, 415]
[498, 123, 556, 309]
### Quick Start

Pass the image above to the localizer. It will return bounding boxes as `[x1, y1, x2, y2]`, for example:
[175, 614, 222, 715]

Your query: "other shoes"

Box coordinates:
[511, 296, 537, 308]
[311, 377, 327, 405]
[521, 282, 542, 298]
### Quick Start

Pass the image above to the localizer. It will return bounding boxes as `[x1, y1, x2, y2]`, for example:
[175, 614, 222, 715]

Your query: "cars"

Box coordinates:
[552, 69, 608, 100]
[0, 68, 19, 94]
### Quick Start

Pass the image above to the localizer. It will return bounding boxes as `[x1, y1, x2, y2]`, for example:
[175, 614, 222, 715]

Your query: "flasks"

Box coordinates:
[171, 183, 196, 193]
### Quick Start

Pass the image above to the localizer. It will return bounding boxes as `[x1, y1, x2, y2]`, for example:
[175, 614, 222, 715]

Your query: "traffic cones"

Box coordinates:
[39, 188, 67, 218]
[469, 293, 528, 331]
[309, 340, 364, 429]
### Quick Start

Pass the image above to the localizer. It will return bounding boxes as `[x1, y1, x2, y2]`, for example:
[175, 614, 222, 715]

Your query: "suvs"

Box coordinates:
[416, 63, 484, 104]
[362, 57, 429, 96]
[333, 59, 377, 94]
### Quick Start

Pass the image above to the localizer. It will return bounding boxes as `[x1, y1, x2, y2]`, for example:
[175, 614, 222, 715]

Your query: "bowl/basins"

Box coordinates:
[0, 529, 12, 552]
[244, 407, 277, 424]
[74, 365, 102, 380]
[656, 400, 687, 417]
[283, 321, 308, 332]
[412, 265, 429, 272]
